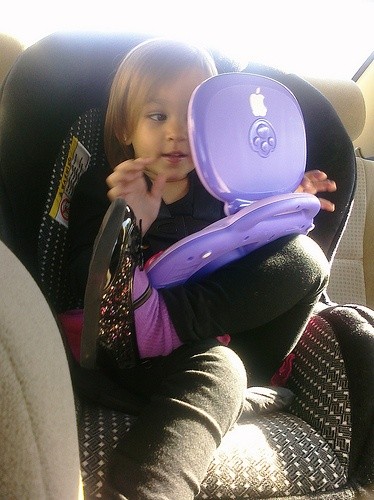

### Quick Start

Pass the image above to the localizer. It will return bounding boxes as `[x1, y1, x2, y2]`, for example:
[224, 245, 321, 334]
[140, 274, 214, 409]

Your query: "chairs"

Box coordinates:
[1, 37, 373, 500]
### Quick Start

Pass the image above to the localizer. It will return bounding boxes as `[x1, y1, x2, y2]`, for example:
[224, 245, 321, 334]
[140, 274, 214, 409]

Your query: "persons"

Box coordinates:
[55, 37, 337, 500]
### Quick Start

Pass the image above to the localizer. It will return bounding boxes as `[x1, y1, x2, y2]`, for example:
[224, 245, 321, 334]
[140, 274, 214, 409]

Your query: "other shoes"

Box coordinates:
[78, 199, 148, 371]
